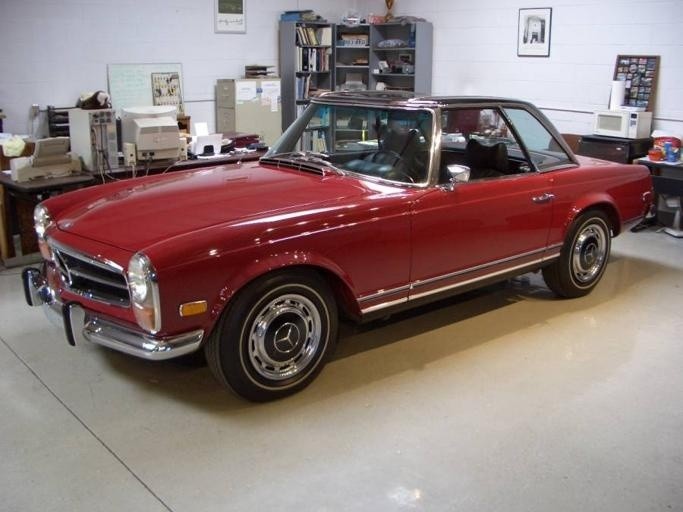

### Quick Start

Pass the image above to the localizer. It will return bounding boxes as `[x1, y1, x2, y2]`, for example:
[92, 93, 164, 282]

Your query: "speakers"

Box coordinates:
[123, 142, 136, 167]
[178, 137, 188, 160]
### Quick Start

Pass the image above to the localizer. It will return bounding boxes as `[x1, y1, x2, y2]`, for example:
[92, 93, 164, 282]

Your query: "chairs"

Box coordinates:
[543, 134, 581, 154]
[365, 130, 508, 179]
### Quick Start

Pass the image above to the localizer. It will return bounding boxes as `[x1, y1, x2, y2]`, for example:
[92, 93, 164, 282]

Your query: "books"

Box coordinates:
[292, 21, 333, 154]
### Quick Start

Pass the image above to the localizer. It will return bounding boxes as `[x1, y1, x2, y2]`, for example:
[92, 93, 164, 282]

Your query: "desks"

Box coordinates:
[0, 150, 270, 257]
[580, 136, 683, 238]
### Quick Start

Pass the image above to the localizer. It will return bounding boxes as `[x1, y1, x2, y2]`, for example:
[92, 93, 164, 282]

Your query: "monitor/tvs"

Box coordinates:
[121, 106, 181, 161]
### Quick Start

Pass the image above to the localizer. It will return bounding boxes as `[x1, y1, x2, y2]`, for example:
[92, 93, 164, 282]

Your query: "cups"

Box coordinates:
[664, 142, 672, 154]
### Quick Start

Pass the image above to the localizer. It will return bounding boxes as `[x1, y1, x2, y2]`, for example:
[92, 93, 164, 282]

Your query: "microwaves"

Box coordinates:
[592, 110, 652, 139]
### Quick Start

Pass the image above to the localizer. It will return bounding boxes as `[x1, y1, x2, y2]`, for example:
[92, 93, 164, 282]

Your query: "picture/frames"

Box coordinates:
[608, 54, 661, 110]
[516, 7, 552, 56]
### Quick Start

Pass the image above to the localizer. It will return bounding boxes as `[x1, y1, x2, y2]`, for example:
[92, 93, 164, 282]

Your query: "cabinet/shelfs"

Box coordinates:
[279, 21, 433, 151]
[217, 78, 282, 150]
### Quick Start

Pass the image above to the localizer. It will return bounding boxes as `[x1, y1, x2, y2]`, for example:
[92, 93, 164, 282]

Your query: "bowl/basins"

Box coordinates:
[649, 149, 662, 160]
[666, 152, 678, 162]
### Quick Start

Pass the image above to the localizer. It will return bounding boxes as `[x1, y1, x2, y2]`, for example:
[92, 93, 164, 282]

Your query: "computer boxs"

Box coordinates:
[67, 109, 119, 171]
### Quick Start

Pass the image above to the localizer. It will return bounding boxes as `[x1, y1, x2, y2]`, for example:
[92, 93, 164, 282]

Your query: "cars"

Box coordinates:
[22, 89, 655, 403]
